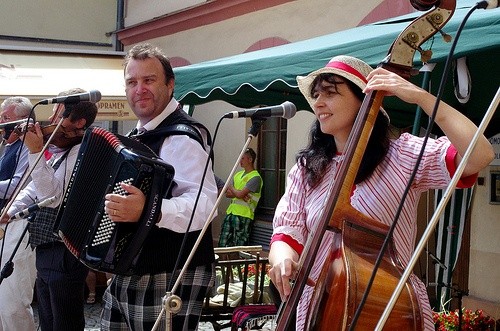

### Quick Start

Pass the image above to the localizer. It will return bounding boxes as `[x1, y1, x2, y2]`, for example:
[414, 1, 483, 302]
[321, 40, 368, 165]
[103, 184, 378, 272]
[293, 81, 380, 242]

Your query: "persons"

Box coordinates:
[0, 96, 37, 331]
[0, 88, 98, 331]
[100, 42, 218, 331]
[214, 148, 264, 261]
[267, 55, 495, 331]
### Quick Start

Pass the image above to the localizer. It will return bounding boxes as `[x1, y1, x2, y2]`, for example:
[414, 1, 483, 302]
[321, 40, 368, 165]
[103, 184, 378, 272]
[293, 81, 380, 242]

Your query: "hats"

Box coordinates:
[296, 55, 390, 127]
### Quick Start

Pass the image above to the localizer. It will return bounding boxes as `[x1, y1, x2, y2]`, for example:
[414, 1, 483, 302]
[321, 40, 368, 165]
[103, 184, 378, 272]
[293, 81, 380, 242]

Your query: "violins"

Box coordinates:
[4, 124, 86, 147]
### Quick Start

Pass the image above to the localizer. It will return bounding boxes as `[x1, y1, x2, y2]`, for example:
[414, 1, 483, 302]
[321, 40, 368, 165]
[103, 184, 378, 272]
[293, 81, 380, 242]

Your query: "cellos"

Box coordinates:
[276, 0, 459, 331]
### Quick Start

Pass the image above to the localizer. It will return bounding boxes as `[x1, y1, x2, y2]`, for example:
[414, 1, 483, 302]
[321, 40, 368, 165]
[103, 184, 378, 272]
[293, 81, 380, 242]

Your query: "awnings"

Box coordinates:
[171, 0, 500, 115]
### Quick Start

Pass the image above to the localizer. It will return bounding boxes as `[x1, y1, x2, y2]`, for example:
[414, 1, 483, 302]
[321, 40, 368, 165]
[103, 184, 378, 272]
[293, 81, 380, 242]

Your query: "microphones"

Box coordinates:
[477, 0, 500, 9]
[38, 90, 102, 105]
[8, 195, 58, 221]
[425, 249, 447, 270]
[223, 101, 296, 119]
[0, 117, 34, 129]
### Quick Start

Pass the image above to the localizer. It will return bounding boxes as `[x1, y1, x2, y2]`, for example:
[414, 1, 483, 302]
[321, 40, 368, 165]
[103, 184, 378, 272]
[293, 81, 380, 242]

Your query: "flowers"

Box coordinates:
[433, 307, 496, 331]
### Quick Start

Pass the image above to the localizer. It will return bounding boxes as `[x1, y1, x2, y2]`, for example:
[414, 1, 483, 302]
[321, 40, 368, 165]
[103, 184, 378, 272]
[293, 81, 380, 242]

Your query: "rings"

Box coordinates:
[113, 209, 116, 216]
